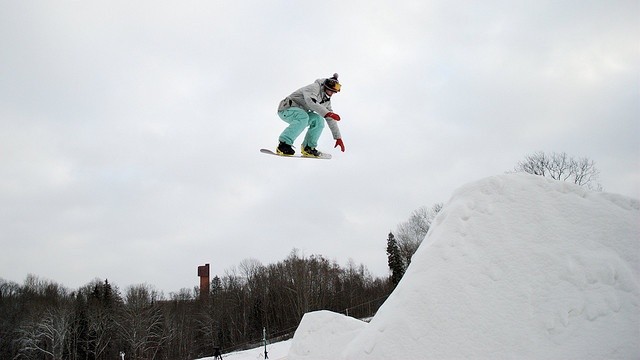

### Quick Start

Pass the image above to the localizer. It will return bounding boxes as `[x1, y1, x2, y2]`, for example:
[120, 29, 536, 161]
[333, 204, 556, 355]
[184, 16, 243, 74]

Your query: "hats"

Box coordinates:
[324, 73, 340, 93]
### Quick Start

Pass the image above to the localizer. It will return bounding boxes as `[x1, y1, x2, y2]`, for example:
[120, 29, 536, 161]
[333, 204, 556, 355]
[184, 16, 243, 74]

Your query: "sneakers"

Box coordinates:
[301, 144, 321, 158]
[276, 142, 295, 156]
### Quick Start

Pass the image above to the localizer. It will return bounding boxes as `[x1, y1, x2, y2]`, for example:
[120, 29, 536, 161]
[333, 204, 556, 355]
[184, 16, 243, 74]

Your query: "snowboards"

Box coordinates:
[260, 149, 332, 159]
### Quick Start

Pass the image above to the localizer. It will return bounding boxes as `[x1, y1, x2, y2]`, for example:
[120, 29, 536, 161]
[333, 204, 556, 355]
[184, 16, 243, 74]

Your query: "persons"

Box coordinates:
[276, 73, 345, 158]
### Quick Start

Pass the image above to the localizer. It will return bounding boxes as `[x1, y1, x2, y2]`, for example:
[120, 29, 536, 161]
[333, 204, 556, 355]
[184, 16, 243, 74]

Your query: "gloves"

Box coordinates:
[326, 112, 340, 121]
[334, 139, 345, 152]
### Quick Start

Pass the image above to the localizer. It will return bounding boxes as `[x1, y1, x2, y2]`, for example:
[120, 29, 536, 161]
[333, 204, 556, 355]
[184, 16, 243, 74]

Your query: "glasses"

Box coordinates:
[333, 82, 341, 92]
